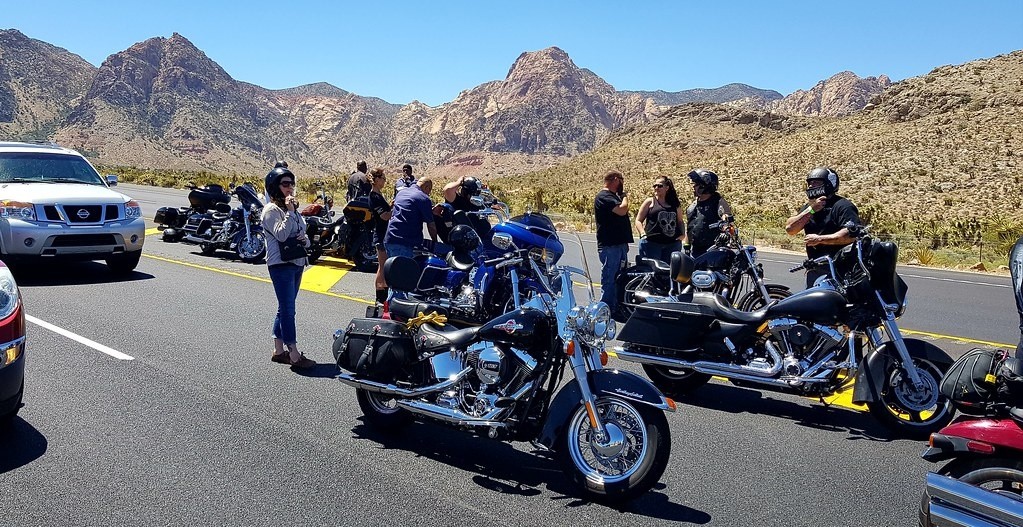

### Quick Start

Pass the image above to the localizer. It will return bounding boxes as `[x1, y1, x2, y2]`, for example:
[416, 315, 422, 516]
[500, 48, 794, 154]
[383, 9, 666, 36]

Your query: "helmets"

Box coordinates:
[265, 168, 295, 199]
[449, 225, 481, 254]
[688, 170, 718, 194]
[275, 161, 288, 168]
[805, 167, 839, 200]
[461, 176, 481, 196]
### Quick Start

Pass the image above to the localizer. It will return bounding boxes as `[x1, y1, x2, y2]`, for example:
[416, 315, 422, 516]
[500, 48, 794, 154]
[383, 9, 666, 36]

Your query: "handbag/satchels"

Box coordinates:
[279, 236, 309, 260]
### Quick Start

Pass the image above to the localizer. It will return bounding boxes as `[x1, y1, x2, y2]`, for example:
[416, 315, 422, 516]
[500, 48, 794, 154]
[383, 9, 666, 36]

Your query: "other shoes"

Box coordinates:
[611, 305, 632, 323]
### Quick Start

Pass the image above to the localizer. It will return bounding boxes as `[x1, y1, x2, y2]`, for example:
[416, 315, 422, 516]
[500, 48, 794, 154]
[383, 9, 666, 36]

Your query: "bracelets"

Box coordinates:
[620, 193, 626, 199]
[684, 244, 690, 246]
[641, 234, 648, 238]
[808, 206, 815, 214]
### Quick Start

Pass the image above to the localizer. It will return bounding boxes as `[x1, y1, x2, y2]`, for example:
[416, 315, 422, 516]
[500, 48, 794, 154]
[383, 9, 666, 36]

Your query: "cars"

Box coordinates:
[0, 258, 24, 423]
[0, 140, 145, 275]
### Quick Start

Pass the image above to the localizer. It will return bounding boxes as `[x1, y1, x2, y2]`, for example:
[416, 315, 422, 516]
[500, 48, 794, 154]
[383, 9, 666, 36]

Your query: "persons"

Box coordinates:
[785, 168, 862, 289]
[260, 167, 316, 368]
[594, 170, 635, 320]
[392, 164, 417, 197]
[635, 176, 687, 264]
[684, 169, 735, 258]
[345, 162, 373, 202]
[383, 177, 437, 302]
[443, 177, 501, 238]
[264, 161, 288, 203]
[368, 169, 396, 302]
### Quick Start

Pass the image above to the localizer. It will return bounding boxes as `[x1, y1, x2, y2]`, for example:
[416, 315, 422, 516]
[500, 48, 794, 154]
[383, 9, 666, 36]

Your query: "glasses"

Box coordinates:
[382, 177, 386, 181]
[280, 180, 295, 188]
[653, 184, 663, 188]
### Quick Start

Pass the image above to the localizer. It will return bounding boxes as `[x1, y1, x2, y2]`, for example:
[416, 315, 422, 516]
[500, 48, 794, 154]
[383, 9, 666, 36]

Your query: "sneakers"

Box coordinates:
[272, 350, 290, 363]
[289, 352, 315, 369]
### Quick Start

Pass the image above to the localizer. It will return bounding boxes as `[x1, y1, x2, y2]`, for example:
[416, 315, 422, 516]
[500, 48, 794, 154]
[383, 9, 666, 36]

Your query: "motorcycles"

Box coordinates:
[300, 181, 564, 327]
[613, 210, 957, 439]
[330, 231, 677, 502]
[153, 181, 237, 242]
[614, 215, 795, 323]
[182, 182, 267, 263]
[917, 347, 1023, 527]
